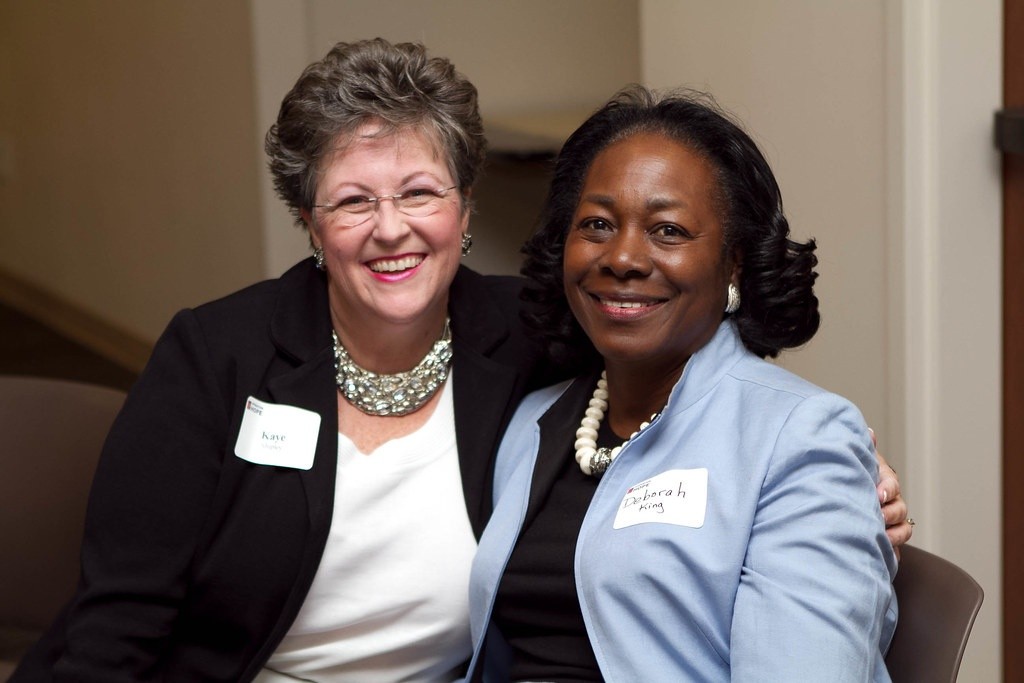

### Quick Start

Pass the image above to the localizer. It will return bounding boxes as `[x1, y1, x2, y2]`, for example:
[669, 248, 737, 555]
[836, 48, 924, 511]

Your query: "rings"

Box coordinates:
[904, 518, 915, 536]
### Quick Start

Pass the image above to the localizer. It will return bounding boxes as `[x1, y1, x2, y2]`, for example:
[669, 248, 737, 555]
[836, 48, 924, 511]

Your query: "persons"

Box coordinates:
[457, 82, 901, 683]
[0, 36, 915, 683]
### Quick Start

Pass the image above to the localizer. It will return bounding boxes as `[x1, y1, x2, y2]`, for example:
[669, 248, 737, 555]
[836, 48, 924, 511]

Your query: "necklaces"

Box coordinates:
[575, 370, 659, 479]
[332, 316, 453, 418]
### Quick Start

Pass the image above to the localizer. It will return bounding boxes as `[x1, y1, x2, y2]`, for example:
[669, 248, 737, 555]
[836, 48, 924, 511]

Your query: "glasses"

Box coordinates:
[309, 181, 461, 230]
